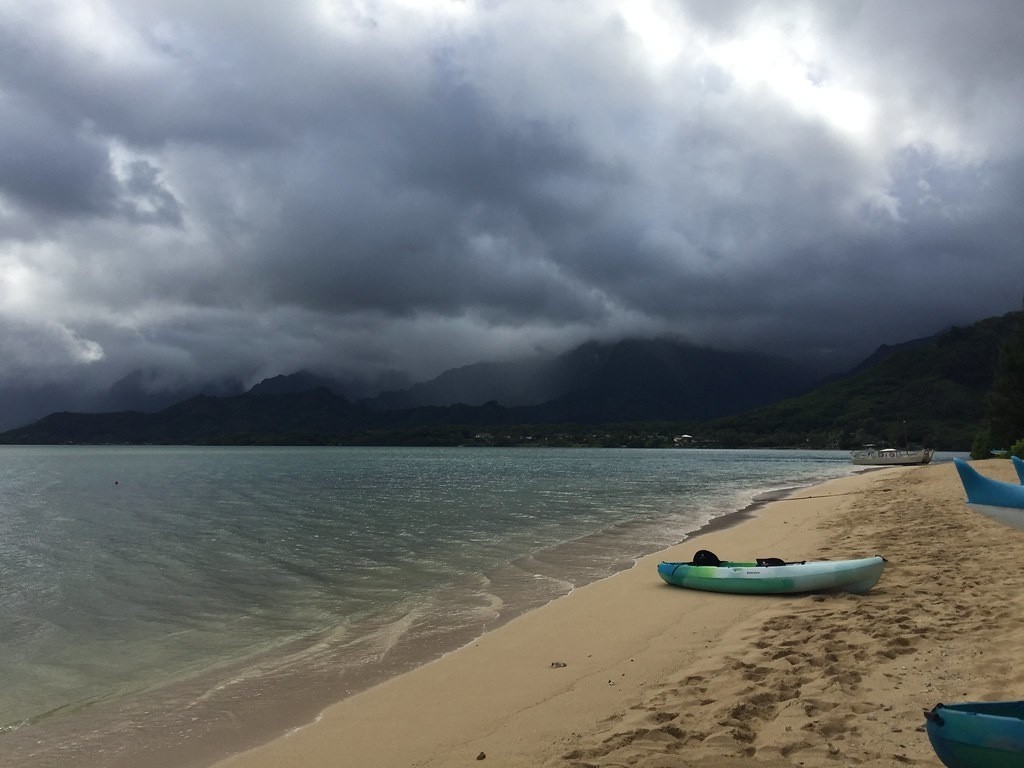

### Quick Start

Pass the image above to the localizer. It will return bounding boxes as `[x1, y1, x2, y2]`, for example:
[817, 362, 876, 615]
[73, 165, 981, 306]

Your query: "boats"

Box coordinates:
[953, 456, 1024, 509]
[925, 700, 1024, 768]
[658, 555, 887, 594]
[849, 443, 936, 466]
